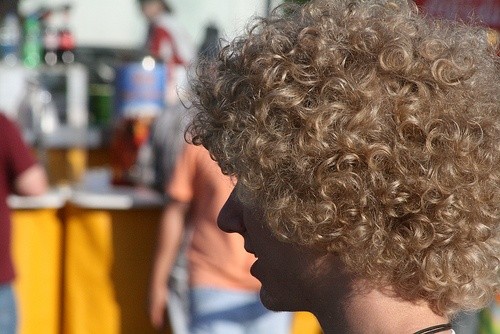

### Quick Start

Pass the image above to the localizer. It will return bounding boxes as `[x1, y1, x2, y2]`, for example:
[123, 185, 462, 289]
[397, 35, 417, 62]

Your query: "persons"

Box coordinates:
[0, 110, 49, 334]
[146, 121, 294, 334]
[173, 1, 500, 334]
[137, 0, 183, 64]
